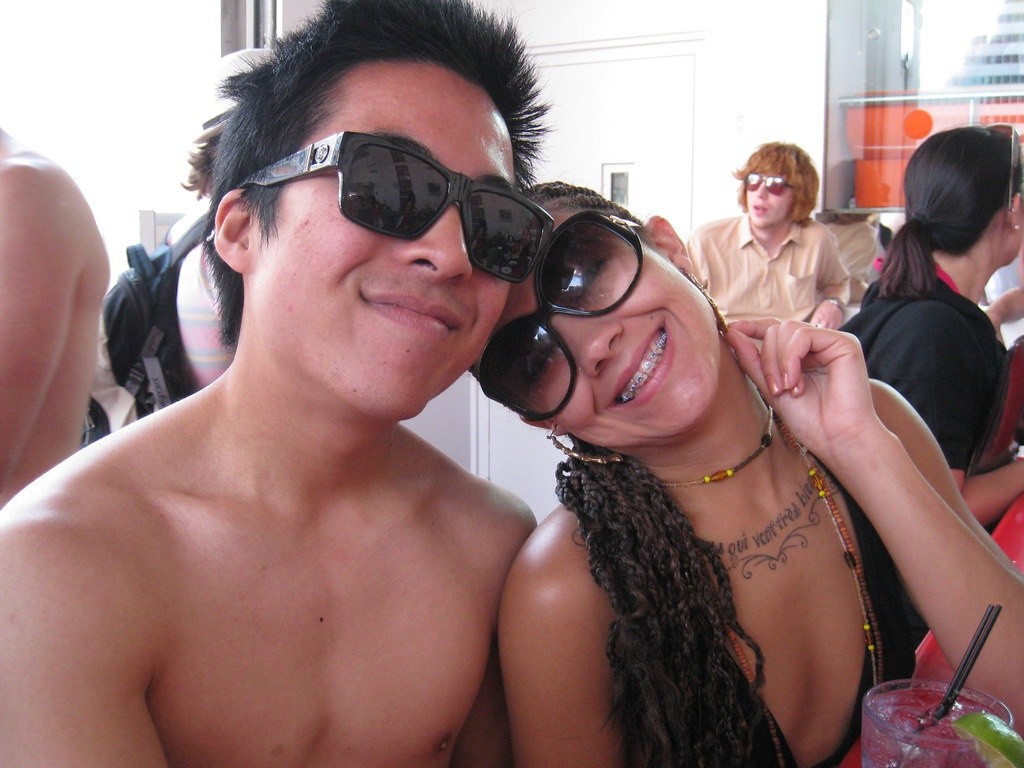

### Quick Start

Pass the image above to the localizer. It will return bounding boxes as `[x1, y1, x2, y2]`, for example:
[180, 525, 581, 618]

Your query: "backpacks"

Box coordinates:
[102, 211, 210, 411]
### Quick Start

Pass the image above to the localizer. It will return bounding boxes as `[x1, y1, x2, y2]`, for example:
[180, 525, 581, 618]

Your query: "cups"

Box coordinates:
[862, 678, 1015, 768]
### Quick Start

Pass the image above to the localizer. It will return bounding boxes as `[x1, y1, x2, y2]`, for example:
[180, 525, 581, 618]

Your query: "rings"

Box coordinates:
[816, 324, 821, 328]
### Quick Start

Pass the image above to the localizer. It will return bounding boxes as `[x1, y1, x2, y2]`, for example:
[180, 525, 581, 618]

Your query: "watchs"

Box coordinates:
[824, 297, 844, 311]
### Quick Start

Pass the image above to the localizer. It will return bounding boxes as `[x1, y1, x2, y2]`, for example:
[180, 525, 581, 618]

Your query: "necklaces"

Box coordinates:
[697, 389, 884, 768]
[655, 407, 773, 488]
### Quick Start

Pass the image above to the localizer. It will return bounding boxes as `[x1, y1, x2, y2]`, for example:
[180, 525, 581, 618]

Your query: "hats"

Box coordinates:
[192, 47, 275, 143]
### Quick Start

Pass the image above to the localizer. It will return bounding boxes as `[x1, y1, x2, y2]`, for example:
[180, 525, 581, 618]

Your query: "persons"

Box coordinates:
[469, 181, 1024, 768]
[819, 160, 889, 303]
[838, 123, 1024, 654]
[0, 0, 553, 768]
[687, 140, 852, 356]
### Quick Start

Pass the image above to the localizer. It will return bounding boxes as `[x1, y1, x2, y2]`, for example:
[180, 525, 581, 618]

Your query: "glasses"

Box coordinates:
[746, 174, 796, 195]
[237, 131, 555, 283]
[988, 124, 1020, 210]
[480, 209, 643, 422]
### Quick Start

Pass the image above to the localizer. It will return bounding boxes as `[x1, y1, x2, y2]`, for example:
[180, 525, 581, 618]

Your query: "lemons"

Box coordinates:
[951, 711, 1024, 768]
